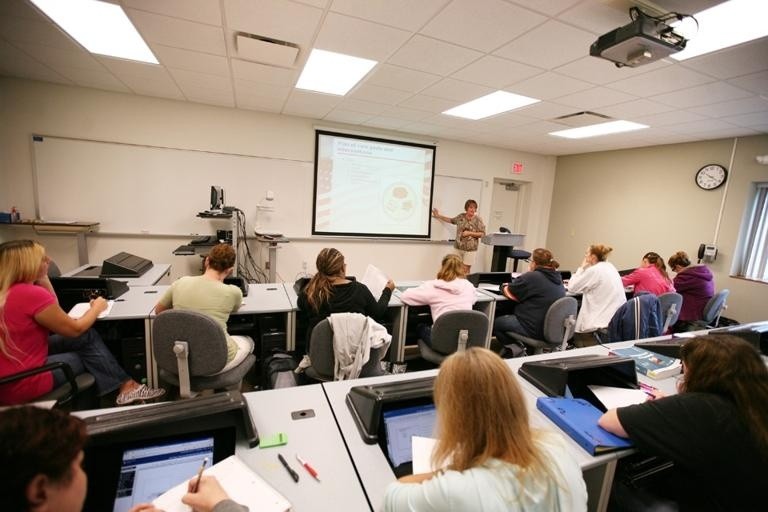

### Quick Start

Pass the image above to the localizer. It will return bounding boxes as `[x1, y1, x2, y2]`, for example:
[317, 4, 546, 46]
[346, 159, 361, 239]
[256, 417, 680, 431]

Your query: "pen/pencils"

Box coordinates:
[643, 391, 656, 399]
[297, 455, 321, 482]
[278, 453, 299, 482]
[193, 459, 208, 494]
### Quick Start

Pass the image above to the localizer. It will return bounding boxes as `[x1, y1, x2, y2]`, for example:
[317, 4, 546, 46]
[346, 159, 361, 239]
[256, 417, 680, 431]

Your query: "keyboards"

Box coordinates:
[192, 236, 210, 243]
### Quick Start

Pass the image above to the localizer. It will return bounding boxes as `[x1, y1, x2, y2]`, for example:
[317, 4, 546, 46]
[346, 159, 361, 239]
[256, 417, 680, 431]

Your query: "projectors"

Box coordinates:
[588, 5, 700, 69]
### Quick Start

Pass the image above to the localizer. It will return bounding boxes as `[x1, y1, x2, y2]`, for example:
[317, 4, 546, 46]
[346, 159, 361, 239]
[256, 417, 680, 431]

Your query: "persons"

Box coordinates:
[400, 253, 477, 356]
[155, 244, 255, 398]
[383, 346, 589, 512]
[432, 199, 486, 273]
[296, 248, 395, 376]
[0, 406, 249, 512]
[0, 239, 165, 405]
[668, 251, 714, 321]
[621, 251, 676, 335]
[493, 248, 566, 358]
[567, 244, 627, 334]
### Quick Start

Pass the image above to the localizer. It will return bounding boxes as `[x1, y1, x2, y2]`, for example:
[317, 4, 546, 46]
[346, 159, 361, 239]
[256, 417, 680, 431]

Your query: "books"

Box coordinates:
[411, 435, 459, 475]
[589, 385, 650, 410]
[608, 345, 682, 380]
[151, 455, 292, 512]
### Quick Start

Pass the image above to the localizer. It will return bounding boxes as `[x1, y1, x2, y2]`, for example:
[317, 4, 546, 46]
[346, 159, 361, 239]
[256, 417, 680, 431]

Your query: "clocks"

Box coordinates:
[695, 164, 728, 190]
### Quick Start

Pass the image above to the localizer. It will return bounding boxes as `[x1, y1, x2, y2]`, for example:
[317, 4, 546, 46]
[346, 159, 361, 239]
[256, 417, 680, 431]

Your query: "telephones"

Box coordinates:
[697, 245, 717, 265]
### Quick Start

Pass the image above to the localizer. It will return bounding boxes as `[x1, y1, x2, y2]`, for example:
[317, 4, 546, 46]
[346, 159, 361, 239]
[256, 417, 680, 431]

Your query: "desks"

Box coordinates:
[389, 282, 494, 370]
[321, 345, 677, 512]
[283, 281, 405, 365]
[0, 218, 101, 267]
[56, 261, 172, 288]
[475, 286, 523, 359]
[53, 276, 169, 410]
[149, 284, 292, 396]
[0, 384, 374, 512]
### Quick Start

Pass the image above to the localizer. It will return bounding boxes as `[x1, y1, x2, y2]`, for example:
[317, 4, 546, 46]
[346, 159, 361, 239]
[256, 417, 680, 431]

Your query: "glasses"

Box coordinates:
[672, 266, 676, 272]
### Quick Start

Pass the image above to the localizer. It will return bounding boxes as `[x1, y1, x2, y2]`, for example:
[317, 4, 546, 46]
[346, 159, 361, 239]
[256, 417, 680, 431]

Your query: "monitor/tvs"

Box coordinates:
[211, 185, 225, 211]
[92, 424, 238, 511]
[376, 398, 438, 480]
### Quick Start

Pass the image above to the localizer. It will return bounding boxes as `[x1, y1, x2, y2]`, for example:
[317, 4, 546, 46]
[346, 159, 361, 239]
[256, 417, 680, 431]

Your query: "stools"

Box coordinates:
[507, 249, 531, 272]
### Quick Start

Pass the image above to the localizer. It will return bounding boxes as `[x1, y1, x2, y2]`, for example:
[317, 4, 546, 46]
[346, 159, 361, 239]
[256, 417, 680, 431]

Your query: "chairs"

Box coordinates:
[418, 310, 490, 374]
[153, 309, 256, 399]
[650, 292, 684, 336]
[1, 358, 97, 414]
[303, 317, 386, 384]
[589, 294, 658, 345]
[667, 288, 731, 335]
[505, 296, 580, 362]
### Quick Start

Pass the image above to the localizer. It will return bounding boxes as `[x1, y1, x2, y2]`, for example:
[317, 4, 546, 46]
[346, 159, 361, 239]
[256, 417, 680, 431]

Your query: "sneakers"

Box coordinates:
[117, 385, 166, 404]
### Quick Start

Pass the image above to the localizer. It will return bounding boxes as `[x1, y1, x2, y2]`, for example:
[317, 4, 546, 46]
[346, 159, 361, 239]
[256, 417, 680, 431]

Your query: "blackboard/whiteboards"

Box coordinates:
[29, 132, 483, 244]
